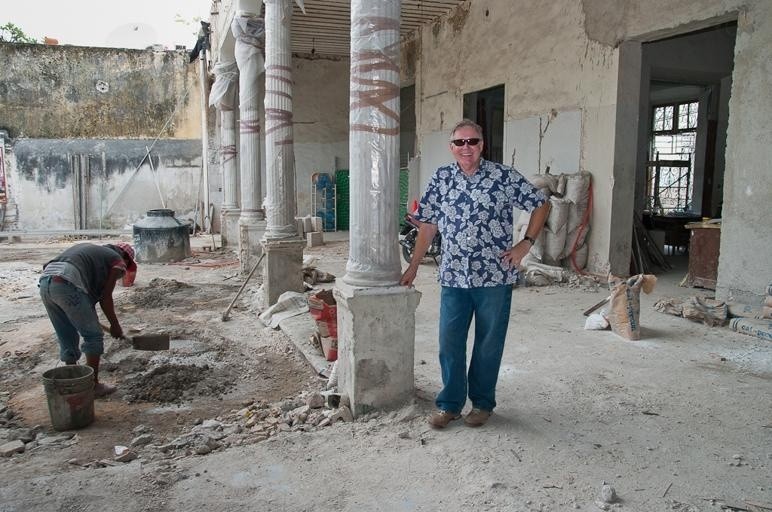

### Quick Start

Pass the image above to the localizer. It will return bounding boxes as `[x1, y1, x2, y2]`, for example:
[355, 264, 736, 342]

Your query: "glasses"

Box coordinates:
[450, 139, 482, 146]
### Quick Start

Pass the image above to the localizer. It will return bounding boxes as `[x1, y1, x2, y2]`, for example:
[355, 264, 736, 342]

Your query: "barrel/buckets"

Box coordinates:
[41, 348, 95, 431]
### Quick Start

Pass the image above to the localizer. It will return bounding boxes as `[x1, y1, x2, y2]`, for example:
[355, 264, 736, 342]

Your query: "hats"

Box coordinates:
[118, 242, 134, 260]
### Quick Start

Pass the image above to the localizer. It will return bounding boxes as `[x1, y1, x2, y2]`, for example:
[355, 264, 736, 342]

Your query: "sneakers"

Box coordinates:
[95, 382, 117, 397]
[464, 407, 493, 427]
[428, 407, 462, 428]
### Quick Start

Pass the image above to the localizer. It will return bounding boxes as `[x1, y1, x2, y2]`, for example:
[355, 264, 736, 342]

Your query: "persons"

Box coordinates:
[399, 118, 550, 429]
[39, 242, 137, 400]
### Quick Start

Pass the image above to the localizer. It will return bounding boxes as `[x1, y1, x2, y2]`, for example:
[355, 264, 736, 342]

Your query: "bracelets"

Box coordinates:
[524, 235, 538, 246]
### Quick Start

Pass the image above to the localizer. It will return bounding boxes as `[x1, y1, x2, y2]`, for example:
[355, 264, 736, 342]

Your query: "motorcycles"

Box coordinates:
[398, 199, 442, 266]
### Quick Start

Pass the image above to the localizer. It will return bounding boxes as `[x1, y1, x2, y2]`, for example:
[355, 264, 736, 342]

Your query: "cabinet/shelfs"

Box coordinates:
[311, 183, 337, 232]
[684, 222, 721, 290]
